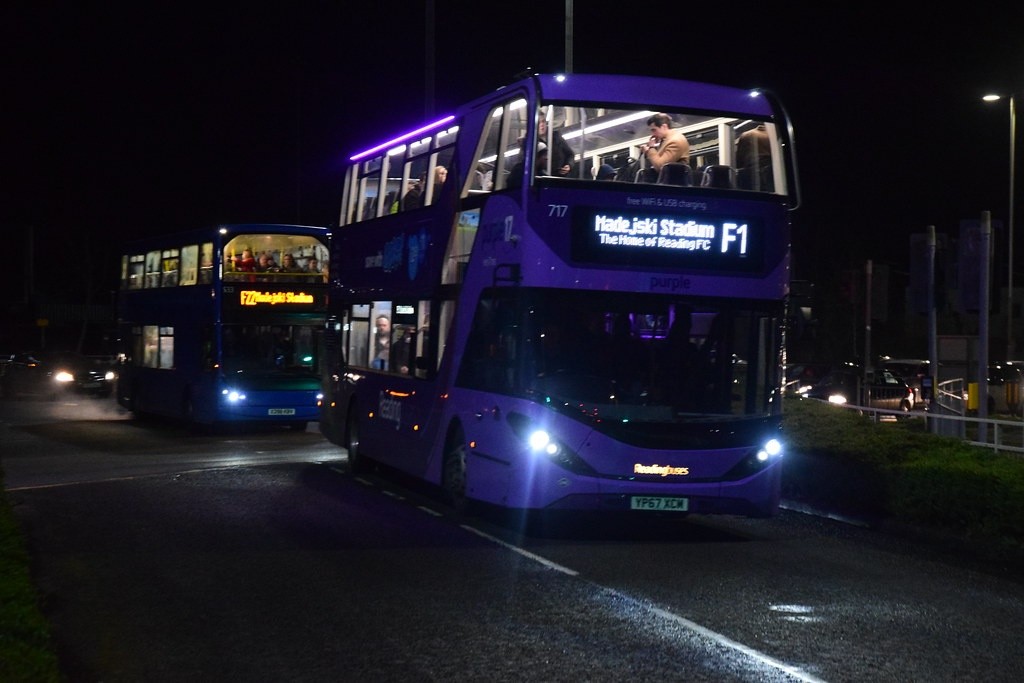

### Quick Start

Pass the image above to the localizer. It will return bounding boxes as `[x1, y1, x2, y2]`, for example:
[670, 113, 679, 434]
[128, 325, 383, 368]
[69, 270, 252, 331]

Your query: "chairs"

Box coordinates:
[349, 150, 777, 223]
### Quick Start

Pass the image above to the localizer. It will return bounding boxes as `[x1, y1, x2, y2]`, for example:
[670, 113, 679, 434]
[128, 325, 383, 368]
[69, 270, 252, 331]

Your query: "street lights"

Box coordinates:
[981, 92, 1015, 361]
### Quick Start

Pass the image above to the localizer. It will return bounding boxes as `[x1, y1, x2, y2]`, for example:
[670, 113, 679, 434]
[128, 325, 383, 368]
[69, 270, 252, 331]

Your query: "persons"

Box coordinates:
[390, 176, 426, 214]
[146, 329, 158, 365]
[596, 164, 617, 181]
[435, 165, 447, 186]
[520, 110, 575, 176]
[374, 315, 390, 371]
[507, 141, 547, 187]
[471, 170, 494, 190]
[639, 113, 689, 174]
[259, 250, 323, 283]
[392, 326, 415, 373]
[231, 249, 257, 282]
[166, 255, 213, 287]
[737, 125, 771, 168]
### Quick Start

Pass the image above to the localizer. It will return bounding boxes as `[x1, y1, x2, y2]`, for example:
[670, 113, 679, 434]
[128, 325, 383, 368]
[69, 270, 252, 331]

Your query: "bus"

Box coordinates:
[116, 223, 331, 430]
[319, 73, 801, 527]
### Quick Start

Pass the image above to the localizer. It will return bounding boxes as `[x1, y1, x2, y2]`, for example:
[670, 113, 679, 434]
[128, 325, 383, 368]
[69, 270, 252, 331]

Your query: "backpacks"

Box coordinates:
[615, 157, 644, 182]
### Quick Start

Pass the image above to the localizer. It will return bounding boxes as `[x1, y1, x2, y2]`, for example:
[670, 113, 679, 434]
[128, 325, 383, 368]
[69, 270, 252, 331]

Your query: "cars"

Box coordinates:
[800, 352, 1024, 420]
[3, 350, 114, 397]
